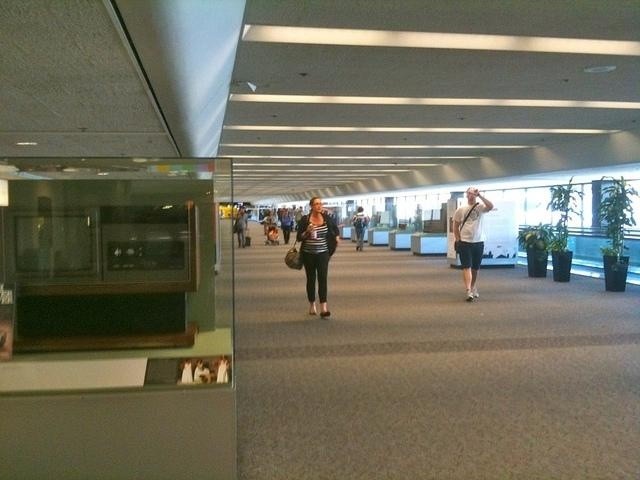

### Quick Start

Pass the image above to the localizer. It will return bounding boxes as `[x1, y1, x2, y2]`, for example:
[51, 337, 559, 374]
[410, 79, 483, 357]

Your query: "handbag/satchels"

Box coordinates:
[454, 241, 461, 253]
[233, 223, 238, 232]
[285, 248, 303, 269]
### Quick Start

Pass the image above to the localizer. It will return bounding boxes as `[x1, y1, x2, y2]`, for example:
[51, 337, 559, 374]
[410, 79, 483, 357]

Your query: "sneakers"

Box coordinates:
[466, 288, 478, 300]
[310, 308, 330, 316]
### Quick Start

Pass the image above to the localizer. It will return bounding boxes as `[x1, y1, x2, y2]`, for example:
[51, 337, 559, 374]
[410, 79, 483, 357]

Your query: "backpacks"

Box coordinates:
[355, 214, 364, 228]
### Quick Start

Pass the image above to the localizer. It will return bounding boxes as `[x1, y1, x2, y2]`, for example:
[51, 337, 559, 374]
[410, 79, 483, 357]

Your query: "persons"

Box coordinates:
[452, 185, 495, 301]
[296, 197, 342, 317]
[258, 205, 308, 245]
[236, 206, 248, 248]
[351, 206, 371, 251]
[181, 355, 230, 383]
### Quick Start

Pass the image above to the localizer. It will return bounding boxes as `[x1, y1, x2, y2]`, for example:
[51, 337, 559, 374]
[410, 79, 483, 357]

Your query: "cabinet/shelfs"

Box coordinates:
[447, 197, 519, 269]
[339, 226, 448, 255]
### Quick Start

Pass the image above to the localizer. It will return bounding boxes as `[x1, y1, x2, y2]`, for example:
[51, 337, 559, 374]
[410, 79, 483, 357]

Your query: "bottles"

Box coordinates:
[310, 221, 317, 240]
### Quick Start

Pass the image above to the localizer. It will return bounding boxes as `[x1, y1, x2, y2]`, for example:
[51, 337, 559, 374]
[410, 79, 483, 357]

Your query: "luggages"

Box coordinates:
[245, 229, 250, 246]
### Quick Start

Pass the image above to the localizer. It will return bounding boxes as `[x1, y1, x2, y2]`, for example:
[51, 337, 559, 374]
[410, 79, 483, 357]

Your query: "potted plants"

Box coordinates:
[515, 175, 640, 292]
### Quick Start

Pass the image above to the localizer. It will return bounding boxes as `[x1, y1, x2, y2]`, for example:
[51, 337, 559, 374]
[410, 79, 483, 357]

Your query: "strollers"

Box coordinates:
[265, 223, 279, 246]
